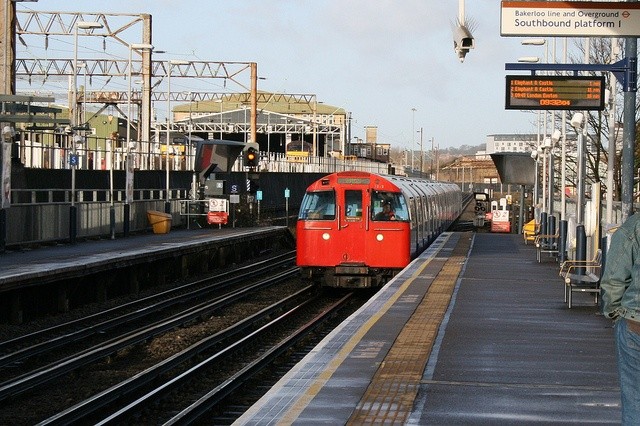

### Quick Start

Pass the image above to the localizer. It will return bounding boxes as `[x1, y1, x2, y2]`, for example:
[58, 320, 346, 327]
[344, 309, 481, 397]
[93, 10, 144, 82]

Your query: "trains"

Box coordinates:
[296, 170, 463, 288]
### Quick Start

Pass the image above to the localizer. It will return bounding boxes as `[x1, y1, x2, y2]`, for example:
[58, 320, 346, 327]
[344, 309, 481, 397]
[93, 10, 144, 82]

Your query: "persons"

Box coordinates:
[599, 210, 639, 425]
[377, 192, 395, 211]
[372, 202, 403, 221]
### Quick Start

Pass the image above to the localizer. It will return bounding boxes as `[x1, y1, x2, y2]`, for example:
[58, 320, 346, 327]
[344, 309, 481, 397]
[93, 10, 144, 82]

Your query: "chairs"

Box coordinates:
[534, 228, 560, 263]
[558, 249, 602, 308]
[524, 223, 541, 245]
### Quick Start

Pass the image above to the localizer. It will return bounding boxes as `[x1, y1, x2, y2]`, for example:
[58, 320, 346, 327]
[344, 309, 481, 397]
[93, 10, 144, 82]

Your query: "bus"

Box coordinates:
[287, 141, 313, 162]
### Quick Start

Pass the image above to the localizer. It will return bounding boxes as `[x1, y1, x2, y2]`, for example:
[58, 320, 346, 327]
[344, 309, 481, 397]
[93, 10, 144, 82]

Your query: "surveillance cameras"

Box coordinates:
[454, 27, 475, 49]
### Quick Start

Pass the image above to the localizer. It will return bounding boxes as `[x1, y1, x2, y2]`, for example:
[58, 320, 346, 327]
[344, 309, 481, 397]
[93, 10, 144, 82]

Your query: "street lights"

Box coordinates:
[123, 44, 154, 237]
[70, 21, 103, 244]
[429, 139, 433, 179]
[215, 100, 223, 139]
[354, 137, 358, 159]
[358, 138, 362, 159]
[412, 108, 415, 172]
[263, 112, 269, 152]
[313, 123, 318, 160]
[280, 117, 287, 158]
[297, 121, 303, 151]
[239, 104, 247, 142]
[165, 59, 190, 213]
[518, 56, 539, 204]
[521, 38, 547, 243]
[417, 128, 422, 176]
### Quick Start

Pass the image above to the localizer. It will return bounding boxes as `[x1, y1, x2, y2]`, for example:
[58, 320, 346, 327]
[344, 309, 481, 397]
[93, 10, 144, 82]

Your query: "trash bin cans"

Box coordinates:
[147, 211, 172, 234]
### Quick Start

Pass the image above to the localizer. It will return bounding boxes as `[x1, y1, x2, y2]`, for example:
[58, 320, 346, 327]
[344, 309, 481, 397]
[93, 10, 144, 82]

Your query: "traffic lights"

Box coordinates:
[244, 143, 259, 166]
